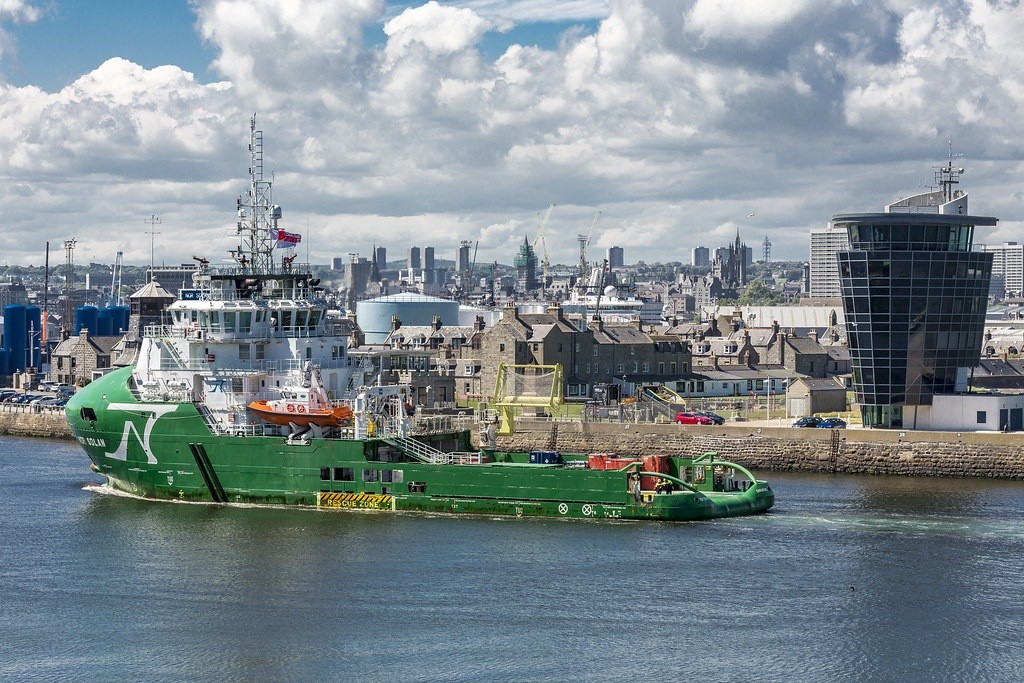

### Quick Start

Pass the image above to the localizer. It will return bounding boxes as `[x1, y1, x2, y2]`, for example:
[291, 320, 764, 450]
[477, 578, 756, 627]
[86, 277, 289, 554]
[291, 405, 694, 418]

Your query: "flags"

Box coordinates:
[277, 230, 302, 248]
[271, 228, 285, 240]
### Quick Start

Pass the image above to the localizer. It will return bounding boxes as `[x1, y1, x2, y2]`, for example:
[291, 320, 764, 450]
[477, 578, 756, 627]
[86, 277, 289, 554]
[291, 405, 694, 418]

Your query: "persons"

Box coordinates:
[632, 474, 641, 503]
[654, 478, 663, 494]
[663, 478, 674, 495]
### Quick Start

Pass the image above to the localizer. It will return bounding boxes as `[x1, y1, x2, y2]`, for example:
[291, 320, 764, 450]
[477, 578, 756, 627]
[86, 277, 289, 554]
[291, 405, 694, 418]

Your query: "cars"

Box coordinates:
[30, 396, 60, 404]
[791, 416, 823, 428]
[817, 418, 847, 429]
[0, 390, 44, 404]
[701, 412, 725, 425]
[675, 411, 711, 425]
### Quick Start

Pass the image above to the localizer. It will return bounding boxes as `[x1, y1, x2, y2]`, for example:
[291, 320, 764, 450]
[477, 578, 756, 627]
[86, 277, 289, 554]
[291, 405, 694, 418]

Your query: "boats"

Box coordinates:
[64, 109, 775, 523]
[248, 385, 353, 425]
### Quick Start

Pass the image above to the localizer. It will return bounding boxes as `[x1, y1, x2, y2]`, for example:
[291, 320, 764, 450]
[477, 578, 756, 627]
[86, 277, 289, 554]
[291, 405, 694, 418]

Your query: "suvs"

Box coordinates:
[52, 383, 76, 391]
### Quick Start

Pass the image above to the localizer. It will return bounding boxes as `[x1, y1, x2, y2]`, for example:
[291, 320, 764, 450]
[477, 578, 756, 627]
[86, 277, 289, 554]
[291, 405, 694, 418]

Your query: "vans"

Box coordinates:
[38, 380, 55, 392]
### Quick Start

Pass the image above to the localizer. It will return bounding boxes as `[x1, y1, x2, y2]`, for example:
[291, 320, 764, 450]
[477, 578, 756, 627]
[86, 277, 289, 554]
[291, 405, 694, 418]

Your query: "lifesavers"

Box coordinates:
[287, 404, 295, 412]
[297, 404, 305, 413]
